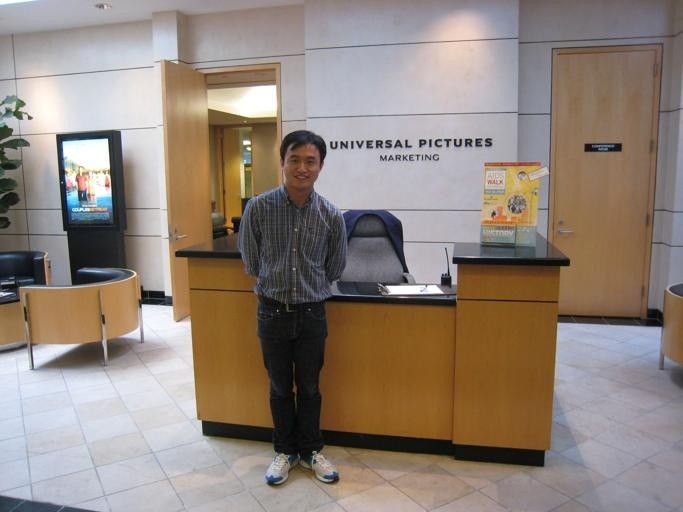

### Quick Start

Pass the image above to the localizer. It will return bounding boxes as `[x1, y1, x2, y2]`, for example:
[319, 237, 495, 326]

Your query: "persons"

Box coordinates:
[237, 130, 348, 487]
[66, 166, 111, 203]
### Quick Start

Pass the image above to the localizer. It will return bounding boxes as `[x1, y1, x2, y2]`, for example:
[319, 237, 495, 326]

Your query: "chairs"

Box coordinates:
[338, 210, 415, 286]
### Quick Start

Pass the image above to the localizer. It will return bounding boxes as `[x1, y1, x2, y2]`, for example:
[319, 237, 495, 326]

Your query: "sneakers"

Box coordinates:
[266, 453, 300, 485]
[300, 451, 339, 482]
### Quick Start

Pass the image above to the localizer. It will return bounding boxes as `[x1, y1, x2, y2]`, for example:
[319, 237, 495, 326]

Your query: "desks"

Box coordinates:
[175, 229, 569, 467]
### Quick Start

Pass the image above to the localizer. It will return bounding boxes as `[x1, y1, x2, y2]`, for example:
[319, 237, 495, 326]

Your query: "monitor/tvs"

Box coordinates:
[56, 129, 128, 231]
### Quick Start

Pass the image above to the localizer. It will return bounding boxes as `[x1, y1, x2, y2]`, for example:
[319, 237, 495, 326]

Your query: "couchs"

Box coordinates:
[658, 281, 683, 374]
[19, 267, 146, 370]
[0, 251, 51, 293]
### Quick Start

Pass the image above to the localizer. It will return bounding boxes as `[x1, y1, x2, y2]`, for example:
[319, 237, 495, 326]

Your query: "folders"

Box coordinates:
[376, 282, 456, 296]
